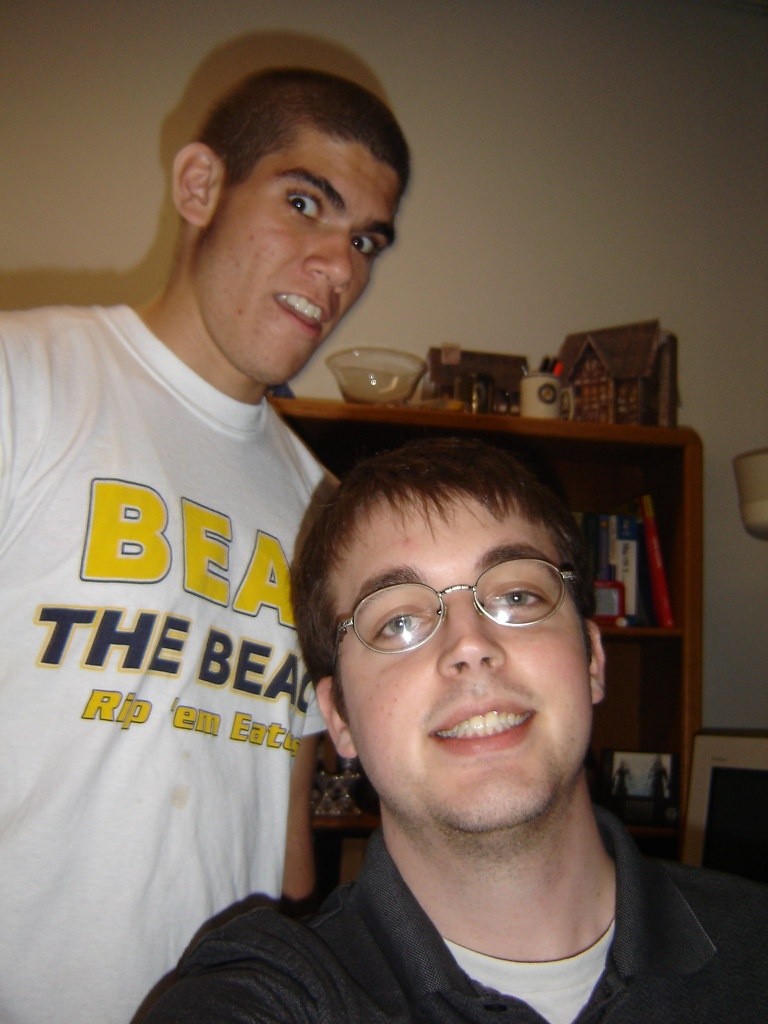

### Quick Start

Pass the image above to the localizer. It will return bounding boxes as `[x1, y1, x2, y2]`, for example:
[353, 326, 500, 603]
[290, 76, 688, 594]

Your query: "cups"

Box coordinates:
[453, 372, 492, 415]
[520, 374, 575, 422]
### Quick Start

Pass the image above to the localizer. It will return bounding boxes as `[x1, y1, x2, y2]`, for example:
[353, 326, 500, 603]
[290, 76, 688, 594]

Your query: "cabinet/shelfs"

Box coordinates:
[267, 399, 708, 898]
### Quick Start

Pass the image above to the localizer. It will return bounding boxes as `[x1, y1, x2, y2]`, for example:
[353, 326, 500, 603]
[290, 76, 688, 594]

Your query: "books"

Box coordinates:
[568, 496, 673, 626]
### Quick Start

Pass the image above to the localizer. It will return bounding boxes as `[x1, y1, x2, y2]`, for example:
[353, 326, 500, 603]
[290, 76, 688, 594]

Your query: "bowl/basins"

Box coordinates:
[327, 348, 428, 408]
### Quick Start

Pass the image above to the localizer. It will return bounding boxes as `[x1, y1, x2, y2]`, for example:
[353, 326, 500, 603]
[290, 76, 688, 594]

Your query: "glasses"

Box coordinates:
[328, 555, 575, 666]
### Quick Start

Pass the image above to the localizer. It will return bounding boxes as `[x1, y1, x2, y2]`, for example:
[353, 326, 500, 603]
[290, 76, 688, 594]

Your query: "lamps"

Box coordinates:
[733, 448, 768, 541]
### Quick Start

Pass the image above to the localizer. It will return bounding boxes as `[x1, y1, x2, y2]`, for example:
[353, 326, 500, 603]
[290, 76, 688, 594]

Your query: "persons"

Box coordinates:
[128, 442, 768, 1024]
[0, 69, 410, 1024]
